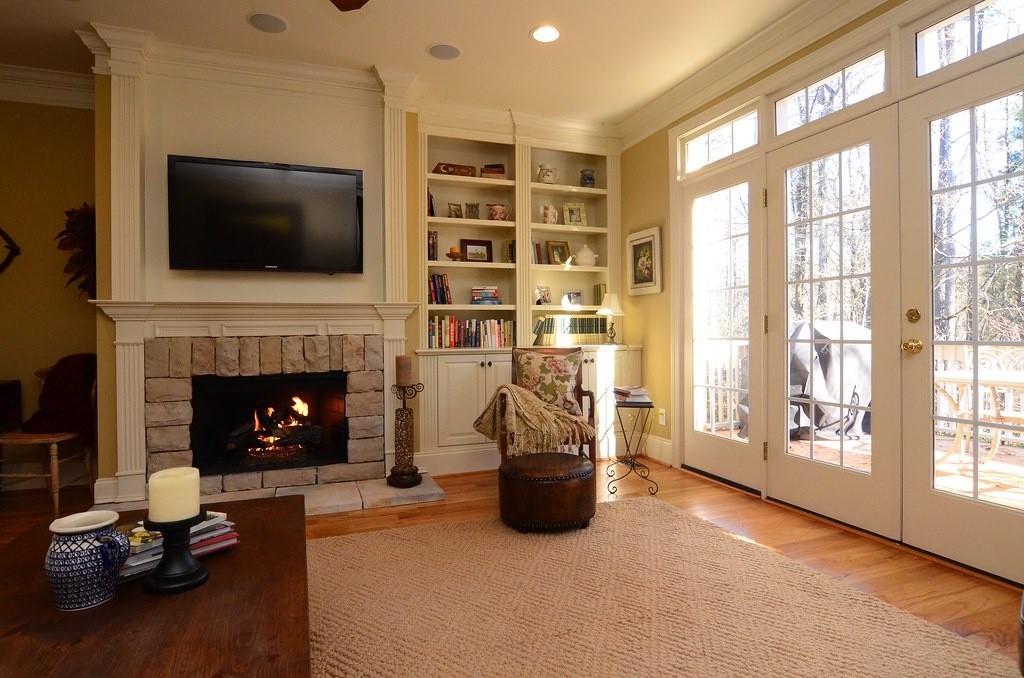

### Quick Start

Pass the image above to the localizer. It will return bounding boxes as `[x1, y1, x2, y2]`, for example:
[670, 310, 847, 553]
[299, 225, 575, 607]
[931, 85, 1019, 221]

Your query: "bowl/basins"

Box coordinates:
[572, 245, 598, 266]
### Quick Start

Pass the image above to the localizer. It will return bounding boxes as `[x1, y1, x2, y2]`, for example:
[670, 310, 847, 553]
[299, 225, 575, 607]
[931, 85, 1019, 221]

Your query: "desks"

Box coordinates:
[0, 495, 310, 678]
[931, 368, 1024, 468]
[606, 402, 659, 494]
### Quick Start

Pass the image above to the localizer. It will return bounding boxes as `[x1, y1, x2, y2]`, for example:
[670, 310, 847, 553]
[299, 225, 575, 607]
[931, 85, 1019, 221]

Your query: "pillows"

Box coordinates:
[512, 349, 585, 416]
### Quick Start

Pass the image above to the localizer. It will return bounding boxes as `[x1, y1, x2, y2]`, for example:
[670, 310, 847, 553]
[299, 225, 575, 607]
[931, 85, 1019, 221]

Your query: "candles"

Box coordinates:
[148, 467, 200, 522]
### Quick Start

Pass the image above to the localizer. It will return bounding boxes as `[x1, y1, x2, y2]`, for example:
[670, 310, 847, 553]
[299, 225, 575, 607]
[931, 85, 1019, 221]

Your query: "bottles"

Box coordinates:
[580, 169, 595, 188]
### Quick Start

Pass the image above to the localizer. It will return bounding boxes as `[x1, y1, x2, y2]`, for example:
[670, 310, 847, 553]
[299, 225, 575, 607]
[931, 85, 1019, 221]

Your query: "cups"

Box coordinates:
[45, 510, 131, 612]
[544, 205, 557, 224]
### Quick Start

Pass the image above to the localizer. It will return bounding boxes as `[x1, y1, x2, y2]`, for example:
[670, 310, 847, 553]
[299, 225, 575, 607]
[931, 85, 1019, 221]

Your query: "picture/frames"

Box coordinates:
[460, 238, 493, 262]
[626, 226, 662, 296]
[563, 202, 587, 226]
[546, 241, 571, 264]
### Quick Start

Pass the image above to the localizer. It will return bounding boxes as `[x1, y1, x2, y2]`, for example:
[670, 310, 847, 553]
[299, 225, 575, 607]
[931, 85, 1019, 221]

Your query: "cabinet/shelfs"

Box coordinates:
[406, 111, 643, 477]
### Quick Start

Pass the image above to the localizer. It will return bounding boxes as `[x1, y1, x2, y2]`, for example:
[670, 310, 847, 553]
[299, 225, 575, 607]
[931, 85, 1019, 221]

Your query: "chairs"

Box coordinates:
[0, 352, 98, 517]
[498, 347, 596, 472]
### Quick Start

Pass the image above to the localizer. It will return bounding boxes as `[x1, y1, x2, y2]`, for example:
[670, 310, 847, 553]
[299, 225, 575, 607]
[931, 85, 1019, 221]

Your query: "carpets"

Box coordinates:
[308, 496, 1022, 678]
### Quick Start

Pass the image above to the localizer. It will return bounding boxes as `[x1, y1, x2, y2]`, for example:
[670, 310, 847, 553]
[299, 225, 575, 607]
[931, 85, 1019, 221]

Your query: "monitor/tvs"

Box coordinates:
[166, 154, 364, 275]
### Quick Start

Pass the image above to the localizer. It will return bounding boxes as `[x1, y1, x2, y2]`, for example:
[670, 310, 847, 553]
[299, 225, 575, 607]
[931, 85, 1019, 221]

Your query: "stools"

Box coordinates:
[498, 453, 596, 535]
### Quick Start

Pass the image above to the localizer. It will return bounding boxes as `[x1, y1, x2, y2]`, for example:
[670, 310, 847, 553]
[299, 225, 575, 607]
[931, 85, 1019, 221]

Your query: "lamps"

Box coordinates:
[596, 293, 624, 345]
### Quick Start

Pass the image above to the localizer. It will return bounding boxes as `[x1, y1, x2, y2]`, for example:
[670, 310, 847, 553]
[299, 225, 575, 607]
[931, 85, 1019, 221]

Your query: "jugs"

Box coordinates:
[538, 164, 559, 183]
[486, 204, 512, 221]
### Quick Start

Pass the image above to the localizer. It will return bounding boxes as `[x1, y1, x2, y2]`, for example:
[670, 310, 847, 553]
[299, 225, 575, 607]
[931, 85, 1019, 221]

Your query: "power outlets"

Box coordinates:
[658, 408, 666, 426]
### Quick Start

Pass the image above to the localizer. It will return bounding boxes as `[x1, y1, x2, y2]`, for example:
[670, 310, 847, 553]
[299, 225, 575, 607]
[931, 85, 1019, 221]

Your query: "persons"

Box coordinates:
[553, 248, 561, 262]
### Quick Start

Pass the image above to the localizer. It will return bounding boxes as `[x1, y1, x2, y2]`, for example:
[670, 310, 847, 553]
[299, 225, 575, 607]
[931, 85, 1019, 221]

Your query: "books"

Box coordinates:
[471, 286, 502, 305]
[428, 315, 516, 348]
[428, 274, 452, 304]
[594, 284, 606, 305]
[116, 511, 240, 577]
[531, 240, 542, 264]
[429, 230, 438, 261]
[427, 186, 436, 216]
[480, 164, 505, 179]
[532, 314, 607, 345]
[614, 385, 652, 403]
[502, 239, 515, 263]
